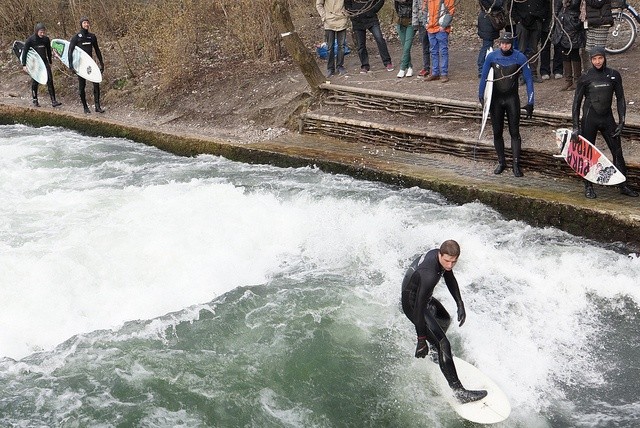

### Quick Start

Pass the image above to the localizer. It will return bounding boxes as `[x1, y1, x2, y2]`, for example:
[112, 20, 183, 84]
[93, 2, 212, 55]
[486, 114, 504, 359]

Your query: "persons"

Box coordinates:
[68, 19, 105, 114]
[551, 0, 586, 91]
[507, 0, 543, 86]
[540, 0, 563, 79]
[22, 23, 62, 107]
[316, 0, 349, 78]
[422, 0, 455, 83]
[401, 240, 487, 404]
[572, 46, 639, 199]
[344, 1, 394, 74]
[503, 0, 520, 37]
[395, 0, 414, 79]
[478, 32, 534, 177]
[478, 0, 506, 77]
[580, 0, 614, 69]
[412, 0, 430, 76]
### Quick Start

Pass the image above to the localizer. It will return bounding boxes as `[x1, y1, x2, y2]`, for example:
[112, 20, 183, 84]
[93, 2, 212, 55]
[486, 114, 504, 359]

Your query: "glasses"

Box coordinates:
[503, 35, 512, 40]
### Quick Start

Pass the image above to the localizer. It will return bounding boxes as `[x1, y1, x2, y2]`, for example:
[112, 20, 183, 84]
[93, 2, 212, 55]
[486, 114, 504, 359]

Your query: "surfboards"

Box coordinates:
[404, 337, 513, 422]
[50, 37, 103, 83]
[12, 40, 49, 86]
[477, 46, 496, 141]
[551, 123, 627, 188]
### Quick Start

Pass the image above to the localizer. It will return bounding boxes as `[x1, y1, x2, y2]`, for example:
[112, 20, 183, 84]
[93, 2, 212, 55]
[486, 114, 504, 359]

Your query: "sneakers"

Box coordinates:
[424, 75, 439, 81]
[542, 74, 550, 79]
[360, 68, 367, 74]
[406, 67, 413, 76]
[397, 70, 405, 78]
[440, 76, 448, 82]
[419, 69, 430, 76]
[555, 73, 563, 79]
[386, 64, 394, 71]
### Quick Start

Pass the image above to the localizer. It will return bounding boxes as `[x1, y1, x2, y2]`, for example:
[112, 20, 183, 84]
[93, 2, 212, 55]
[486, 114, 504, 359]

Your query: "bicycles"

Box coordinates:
[604, 1, 640, 54]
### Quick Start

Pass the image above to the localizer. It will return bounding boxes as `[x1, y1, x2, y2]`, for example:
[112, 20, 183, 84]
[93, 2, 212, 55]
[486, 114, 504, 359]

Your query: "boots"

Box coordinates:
[567, 61, 581, 92]
[559, 61, 573, 91]
